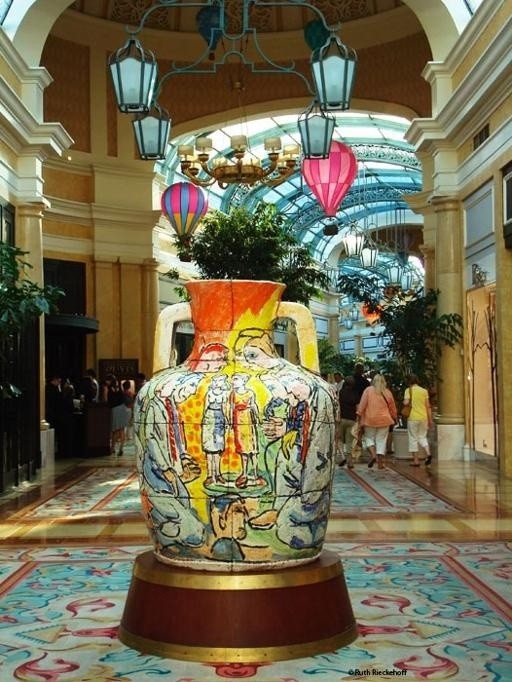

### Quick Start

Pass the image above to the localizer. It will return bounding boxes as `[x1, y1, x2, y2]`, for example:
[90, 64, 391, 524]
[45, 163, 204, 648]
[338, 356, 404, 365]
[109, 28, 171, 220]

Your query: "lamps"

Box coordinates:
[342, 163, 379, 269]
[107, 1, 356, 189]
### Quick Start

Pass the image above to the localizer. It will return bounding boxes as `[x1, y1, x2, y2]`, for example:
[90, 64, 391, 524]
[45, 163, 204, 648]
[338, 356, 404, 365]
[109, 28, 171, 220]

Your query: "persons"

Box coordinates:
[350, 363, 370, 405]
[354, 372, 398, 470]
[332, 372, 345, 395]
[402, 374, 433, 467]
[47, 366, 145, 464]
[334, 375, 360, 470]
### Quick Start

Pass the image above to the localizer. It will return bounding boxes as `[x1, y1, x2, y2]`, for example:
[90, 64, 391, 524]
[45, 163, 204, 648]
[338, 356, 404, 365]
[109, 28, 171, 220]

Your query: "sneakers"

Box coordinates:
[339, 459, 354, 468]
[409, 455, 432, 466]
[368, 456, 385, 469]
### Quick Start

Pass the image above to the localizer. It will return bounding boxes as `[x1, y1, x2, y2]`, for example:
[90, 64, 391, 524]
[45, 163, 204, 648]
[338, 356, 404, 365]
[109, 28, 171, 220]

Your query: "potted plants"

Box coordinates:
[378, 288, 464, 460]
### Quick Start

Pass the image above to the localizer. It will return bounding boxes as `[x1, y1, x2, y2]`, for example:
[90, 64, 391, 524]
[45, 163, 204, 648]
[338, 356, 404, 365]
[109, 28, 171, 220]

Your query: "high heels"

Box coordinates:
[112, 446, 123, 456]
